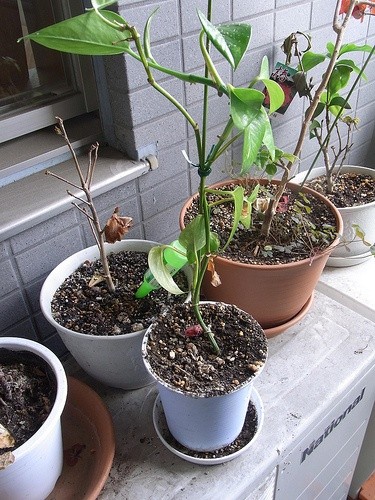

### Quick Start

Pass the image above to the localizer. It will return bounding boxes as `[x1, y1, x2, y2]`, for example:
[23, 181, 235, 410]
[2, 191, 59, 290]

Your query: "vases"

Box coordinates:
[0, 338, 115, 500]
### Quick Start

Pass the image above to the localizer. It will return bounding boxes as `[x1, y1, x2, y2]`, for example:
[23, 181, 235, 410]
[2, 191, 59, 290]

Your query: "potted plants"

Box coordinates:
[16, 0, 300, 466]
[39, 115, 194, 390]
[280, 29, 375, 267]
[179, 0, 375, 339]
